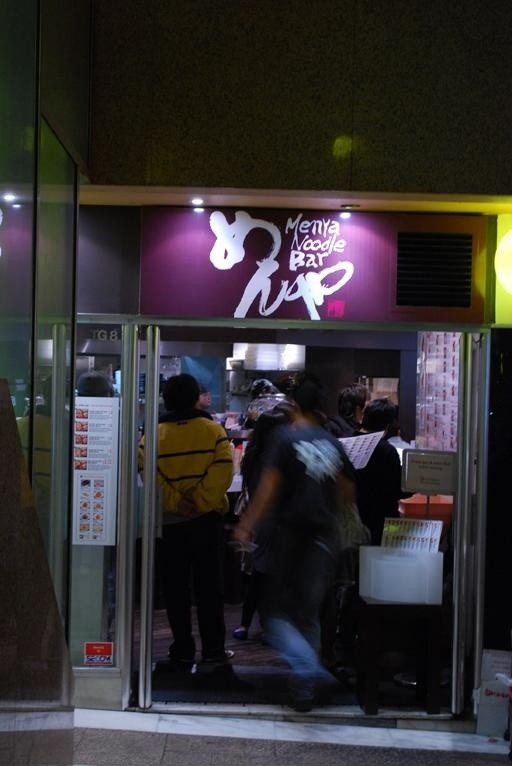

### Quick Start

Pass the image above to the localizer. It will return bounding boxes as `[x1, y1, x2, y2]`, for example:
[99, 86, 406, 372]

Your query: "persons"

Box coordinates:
[75, 371, 119, 640]
[229, 367, 412, 712]
[192, 382, 214, 413]
[139, 372, 235, 663]
[10, 373, 51, 575]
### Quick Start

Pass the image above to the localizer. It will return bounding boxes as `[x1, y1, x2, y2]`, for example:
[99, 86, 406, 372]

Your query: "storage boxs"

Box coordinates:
[355, 543, 448, 609]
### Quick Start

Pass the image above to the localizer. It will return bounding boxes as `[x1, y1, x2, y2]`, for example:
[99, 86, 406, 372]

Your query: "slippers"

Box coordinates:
[203, 649, 235, 662]
[168, 651, 196, 664]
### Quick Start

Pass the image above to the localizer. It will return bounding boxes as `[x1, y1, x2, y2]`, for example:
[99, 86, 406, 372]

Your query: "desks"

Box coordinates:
[354, 593, 455, 720]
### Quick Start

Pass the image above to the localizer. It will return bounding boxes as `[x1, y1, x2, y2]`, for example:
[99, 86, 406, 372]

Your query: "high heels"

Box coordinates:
[232, 628, 248, 640]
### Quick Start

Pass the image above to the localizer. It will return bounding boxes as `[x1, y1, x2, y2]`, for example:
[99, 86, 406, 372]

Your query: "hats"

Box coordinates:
[77, 372, 113, 395]
[198, 383, 211, 394]
[248, 379, 272, 396]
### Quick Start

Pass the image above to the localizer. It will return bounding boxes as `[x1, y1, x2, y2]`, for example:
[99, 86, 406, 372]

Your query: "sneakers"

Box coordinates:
[285, 692, 314, 713]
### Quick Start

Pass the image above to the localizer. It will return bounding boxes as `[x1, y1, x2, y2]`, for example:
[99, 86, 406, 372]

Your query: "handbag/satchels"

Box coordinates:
[335, 502, 372, 553]
